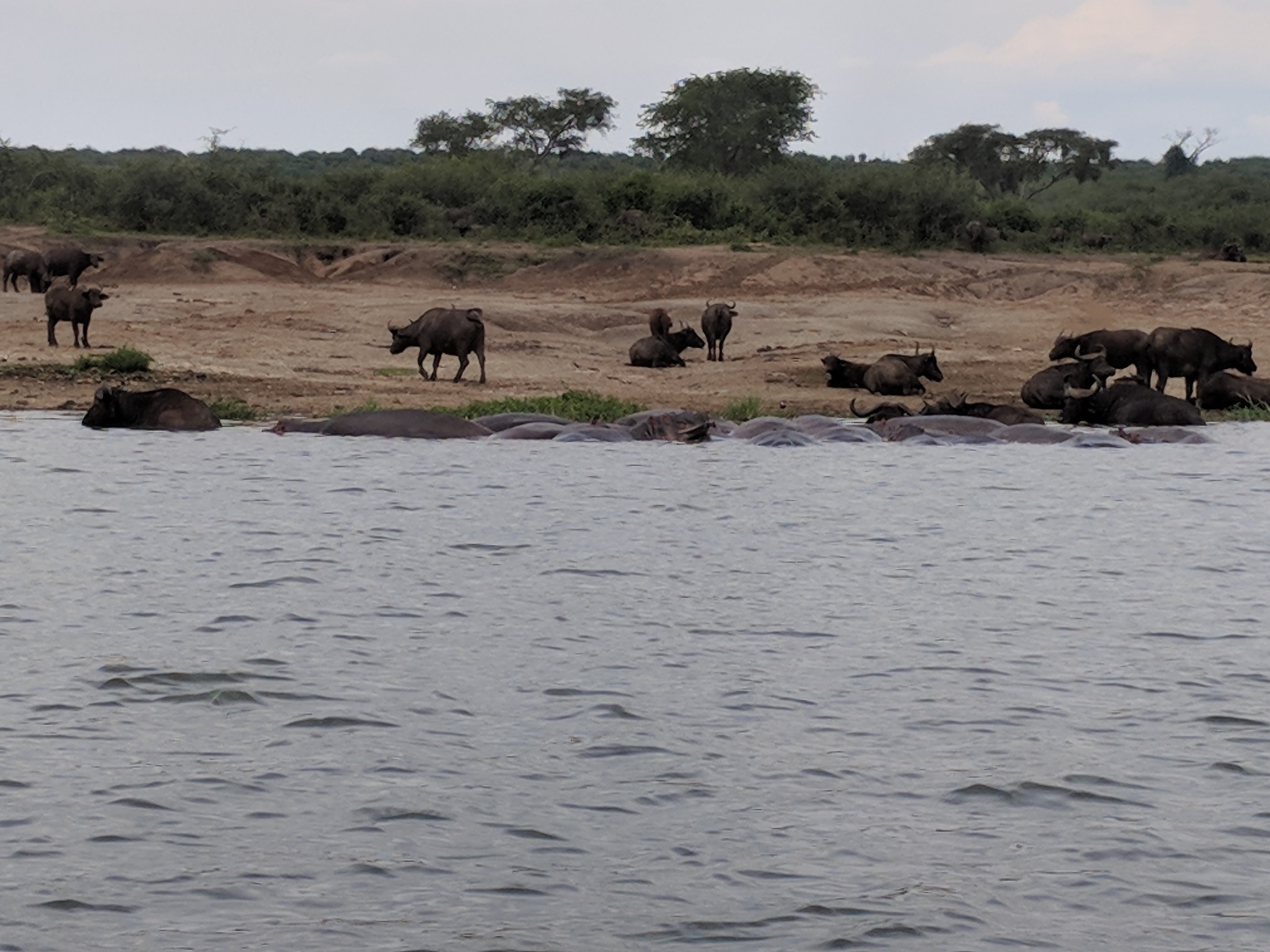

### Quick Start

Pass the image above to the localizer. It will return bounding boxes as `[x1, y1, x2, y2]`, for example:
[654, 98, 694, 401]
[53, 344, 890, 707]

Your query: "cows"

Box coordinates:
[821, 353, 871, 391]
[864, 340, 945, 396]
[1081, 233, 1112, 250]
[629, 319, 706, 367]
[81, 386, 221, 432]
[701, 299, 738, 363]
[1216, 242, 1247, 262]
[44, 247, 107, 292]
[42, 284, 111, 348]
[386, 307, 487, 385]
[4, 250, 58, 294]
[848, 388, 1047, 428]
[649, 307, 672, 336]
[1024, 326, 1270, 426]
[967, 220, 1000, 253]
[1050, 227, 1063, 244]
